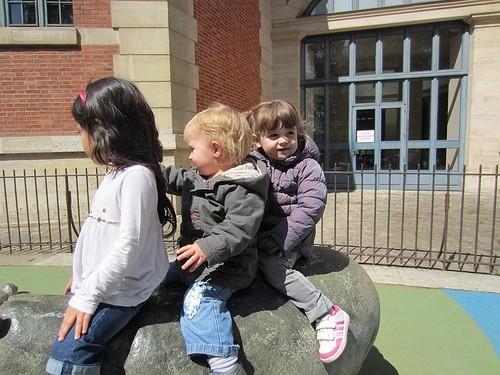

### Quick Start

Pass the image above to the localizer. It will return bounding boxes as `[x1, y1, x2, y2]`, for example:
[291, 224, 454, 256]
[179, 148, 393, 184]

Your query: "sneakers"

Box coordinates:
[315, 306, 349, 364]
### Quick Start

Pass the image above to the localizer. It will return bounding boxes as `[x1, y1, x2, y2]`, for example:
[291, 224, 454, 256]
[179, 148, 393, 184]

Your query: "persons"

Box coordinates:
[237, 100, 351, 362]
[156, 102, 270, 375]
[45, 75, 172, 375]
